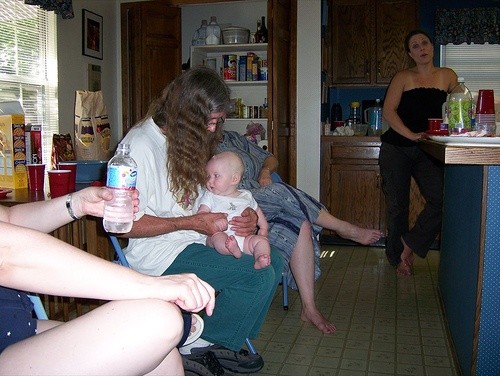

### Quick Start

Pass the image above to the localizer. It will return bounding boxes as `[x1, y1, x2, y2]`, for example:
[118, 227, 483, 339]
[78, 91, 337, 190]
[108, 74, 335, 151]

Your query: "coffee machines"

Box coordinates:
[361, 99, 385, 124]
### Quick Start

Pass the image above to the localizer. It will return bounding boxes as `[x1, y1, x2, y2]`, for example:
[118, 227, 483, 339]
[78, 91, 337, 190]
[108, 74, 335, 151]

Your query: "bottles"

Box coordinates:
[448, 77, 472, 136]
[102, 144, 138, 233]
[331, 103, 343, 121]
[368, 107, 383, 135]
[191, 15, 268, 118]
[348, 102, 360, 121]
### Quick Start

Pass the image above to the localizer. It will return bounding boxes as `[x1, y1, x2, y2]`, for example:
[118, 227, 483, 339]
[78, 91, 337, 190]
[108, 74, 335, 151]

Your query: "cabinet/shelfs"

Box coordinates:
[321, 136, 440, 244]
[327, 0, 420, 88]
[190, 44, 272, 155]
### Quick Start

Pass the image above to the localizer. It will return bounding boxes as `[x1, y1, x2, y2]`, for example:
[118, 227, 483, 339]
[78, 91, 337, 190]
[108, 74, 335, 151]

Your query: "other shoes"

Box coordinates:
[185, 342, 264, 374]
[179, 344, 227, 376]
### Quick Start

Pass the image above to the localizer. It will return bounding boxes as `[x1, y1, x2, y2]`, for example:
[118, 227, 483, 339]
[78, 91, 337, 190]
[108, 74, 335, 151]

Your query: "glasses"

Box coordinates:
[207, 116, 226, 124]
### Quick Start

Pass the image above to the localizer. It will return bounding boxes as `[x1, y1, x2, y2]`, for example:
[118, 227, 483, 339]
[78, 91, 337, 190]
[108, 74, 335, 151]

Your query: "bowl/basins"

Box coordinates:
[58, 160, 108, 181]
[221, 26, 249, 45]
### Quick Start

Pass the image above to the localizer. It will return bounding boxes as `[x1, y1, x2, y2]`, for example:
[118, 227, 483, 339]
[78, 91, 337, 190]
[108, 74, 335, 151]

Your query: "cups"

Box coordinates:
[58, 163, 77, 194]
[334, 120, 368, 136]
[427, 89, 496, 138]
[48, 170, 71, 200]
[26, 165, 46, 191]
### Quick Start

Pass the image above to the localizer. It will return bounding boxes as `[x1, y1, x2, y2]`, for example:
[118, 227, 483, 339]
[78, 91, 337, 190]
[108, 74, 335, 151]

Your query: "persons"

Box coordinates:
[379, 29, 463, 278]
[0, 70, 383, 376]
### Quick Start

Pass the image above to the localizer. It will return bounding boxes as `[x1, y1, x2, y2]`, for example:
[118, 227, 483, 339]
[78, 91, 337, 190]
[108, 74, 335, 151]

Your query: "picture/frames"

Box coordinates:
[82, 9, 104, 61]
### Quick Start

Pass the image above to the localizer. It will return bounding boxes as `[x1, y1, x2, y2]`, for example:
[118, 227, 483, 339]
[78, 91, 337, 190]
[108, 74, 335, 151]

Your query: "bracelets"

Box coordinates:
[66, 193, 78, 219]
